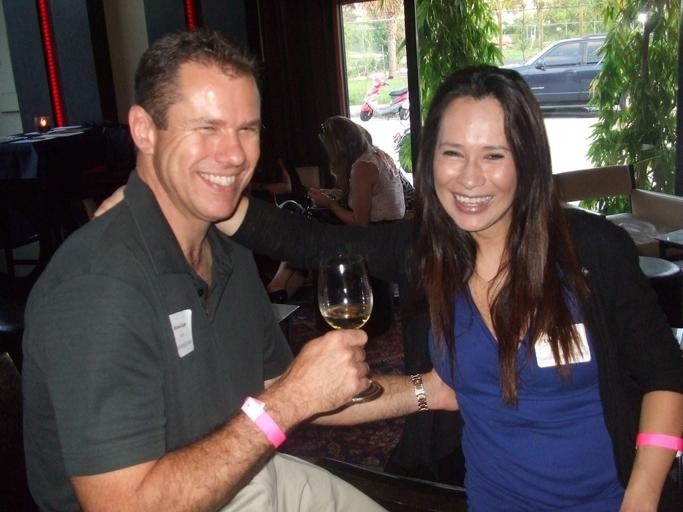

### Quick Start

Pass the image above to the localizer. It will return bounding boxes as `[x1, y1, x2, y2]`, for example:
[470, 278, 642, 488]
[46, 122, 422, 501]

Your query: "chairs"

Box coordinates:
[279, 156, 682, 360]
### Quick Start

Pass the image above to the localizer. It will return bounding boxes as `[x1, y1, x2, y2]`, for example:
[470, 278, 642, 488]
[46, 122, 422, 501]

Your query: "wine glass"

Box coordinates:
[317, 256, 381, 403]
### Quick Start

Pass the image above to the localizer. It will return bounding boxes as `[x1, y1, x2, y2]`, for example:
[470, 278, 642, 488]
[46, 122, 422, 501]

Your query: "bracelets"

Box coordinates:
[239, 395, 291, 452]
[406, 372, 431, 417]
[628, 430, 683, 457]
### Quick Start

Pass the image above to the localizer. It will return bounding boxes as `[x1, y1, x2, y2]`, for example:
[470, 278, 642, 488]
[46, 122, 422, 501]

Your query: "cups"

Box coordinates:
[35, 116, 51, 134]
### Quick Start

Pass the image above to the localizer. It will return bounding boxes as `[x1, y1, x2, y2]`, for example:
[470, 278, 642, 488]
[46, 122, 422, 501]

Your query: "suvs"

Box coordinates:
[508, 32, 631, 112]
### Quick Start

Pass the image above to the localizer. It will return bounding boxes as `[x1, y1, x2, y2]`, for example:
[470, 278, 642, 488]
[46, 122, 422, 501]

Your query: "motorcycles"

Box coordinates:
[354, 73, 410, 124]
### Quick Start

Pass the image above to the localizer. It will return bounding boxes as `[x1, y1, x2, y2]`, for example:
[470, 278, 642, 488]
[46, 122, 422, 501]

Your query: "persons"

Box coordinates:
[360, 128, 418, 212]
[91, 64, 683, 512]
[17, 33, 460, 512]
[261, 113, 408, 304]
[243, 124, 303, 214]
